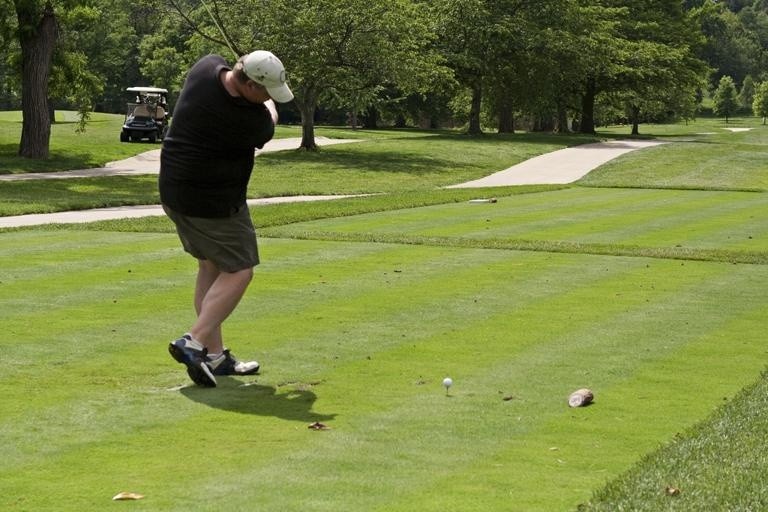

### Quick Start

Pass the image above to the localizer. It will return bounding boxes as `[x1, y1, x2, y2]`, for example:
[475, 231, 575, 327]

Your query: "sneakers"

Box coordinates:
[169, 332, 217, 387]
[206, 348, 259, 374]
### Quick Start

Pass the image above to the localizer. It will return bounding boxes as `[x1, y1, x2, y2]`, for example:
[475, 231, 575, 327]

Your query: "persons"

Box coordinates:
[160, 47, 298, 388]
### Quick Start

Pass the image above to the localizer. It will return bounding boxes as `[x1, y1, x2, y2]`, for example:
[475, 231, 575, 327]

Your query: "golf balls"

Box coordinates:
[442, 378, 452, 387]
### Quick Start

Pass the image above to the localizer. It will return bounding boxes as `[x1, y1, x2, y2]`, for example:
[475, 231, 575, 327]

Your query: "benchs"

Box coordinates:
[133, 105, 165, 119]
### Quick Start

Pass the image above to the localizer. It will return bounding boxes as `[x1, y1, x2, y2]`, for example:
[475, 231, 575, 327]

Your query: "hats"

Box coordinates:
[242, 50, 295, 103]
